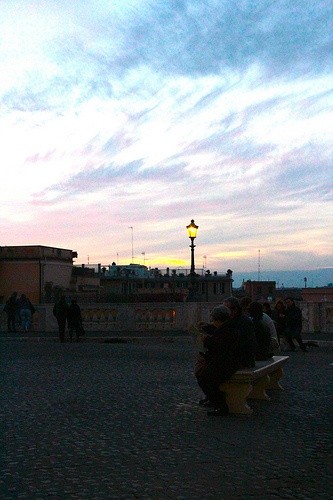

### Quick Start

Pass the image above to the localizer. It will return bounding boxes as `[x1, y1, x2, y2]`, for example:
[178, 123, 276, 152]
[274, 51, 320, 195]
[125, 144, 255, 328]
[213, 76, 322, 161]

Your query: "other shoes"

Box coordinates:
[203, 400, 218, 407]
[289, 348, 298, 351]
[207, 407, 225, 416]
[302, 346, 306, 352]
[199, 398, 207, 404]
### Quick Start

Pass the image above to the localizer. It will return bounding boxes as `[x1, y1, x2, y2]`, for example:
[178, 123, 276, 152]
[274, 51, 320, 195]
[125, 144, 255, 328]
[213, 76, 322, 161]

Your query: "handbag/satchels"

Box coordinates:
[78, 326, 85, 336]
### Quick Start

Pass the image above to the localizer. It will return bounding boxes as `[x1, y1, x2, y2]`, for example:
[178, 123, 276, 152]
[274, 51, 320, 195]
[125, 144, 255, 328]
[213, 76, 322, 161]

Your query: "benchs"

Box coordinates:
[219, 355, 290, 416]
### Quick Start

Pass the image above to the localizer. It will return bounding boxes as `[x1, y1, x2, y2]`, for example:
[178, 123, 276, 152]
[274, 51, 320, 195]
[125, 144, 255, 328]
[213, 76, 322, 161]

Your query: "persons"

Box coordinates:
[4, 292, 18, 333]
[193, 295, 287, 407]
[197, 305, 244, 415]
[67, 299, 82, 343]
[284, 296, 307, 353]
[53, 296, 68, 343]
[18, 294, 33, 334]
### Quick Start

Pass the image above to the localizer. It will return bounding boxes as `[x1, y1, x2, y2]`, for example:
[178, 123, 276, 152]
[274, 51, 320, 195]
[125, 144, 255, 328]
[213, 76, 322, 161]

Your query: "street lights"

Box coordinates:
[186, 220, 200, 302]
